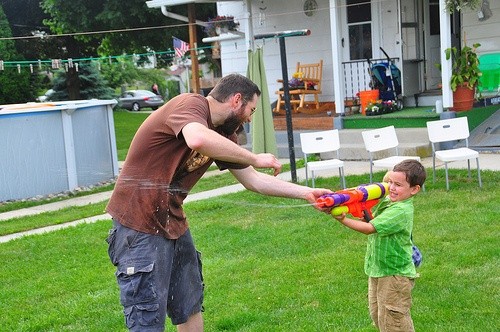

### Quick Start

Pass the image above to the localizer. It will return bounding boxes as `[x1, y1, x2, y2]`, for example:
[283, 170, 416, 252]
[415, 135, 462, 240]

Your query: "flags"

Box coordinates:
[173, 36, 190, 58]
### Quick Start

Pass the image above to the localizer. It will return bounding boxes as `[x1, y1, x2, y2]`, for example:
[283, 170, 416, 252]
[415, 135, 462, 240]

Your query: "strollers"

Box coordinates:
[367, 47, 404, 111]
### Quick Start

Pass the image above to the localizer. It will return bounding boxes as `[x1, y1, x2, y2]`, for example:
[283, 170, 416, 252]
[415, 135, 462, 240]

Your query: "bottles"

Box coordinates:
[435, 100, 443, 113]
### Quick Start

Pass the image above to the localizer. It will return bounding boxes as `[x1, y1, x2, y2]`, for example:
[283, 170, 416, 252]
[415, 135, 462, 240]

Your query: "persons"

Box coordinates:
[152, 82, 159, 95]
[332, 160, 426, 332]
[106, 73, 334, 332]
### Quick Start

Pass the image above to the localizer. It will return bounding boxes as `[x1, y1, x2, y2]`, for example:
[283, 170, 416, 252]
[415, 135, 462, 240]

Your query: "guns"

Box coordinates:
[315, 179, 388, 223]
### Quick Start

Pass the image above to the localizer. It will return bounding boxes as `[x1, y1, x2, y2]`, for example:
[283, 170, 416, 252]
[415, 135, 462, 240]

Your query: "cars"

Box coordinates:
[115, 90, 164, 112]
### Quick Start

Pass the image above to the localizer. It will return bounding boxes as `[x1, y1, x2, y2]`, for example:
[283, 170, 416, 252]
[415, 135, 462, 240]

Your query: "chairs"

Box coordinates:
[426, 116, 482, 190]
[272, 60, 331, 114]
[362, 125, 426, 193]
[300, 129, 347, 192]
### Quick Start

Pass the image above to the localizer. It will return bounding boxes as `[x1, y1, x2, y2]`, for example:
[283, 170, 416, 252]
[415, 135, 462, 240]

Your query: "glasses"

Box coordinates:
[241, 96, 257, 116]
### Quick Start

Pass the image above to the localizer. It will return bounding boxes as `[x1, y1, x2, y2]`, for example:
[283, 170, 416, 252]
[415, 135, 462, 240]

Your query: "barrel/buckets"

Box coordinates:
[360, 89, 379, 115]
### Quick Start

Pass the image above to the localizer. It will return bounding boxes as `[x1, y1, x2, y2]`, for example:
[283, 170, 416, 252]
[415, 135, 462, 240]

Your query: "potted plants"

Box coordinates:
[434, 42, 483, 111]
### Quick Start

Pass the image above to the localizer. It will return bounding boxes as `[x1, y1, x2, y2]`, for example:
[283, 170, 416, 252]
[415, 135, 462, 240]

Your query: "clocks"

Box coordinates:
[303, 0, 319, 17]
